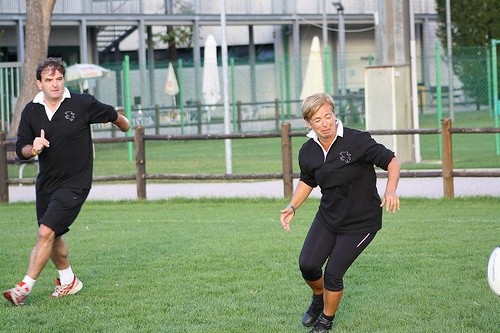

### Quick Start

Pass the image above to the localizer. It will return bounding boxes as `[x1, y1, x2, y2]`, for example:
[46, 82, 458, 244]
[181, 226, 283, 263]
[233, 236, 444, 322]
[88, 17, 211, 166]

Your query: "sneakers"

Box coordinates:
[3, 282, 30, 307]
[308, 311, 335, 333]
[302, 292, 324, 327]
[50, 275, 83, 300]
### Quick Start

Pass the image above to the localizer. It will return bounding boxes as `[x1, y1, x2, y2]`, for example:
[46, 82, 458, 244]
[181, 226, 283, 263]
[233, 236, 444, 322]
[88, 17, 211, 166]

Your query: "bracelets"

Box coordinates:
[32, 149, 38, 156]
[287, 205, 296, 216]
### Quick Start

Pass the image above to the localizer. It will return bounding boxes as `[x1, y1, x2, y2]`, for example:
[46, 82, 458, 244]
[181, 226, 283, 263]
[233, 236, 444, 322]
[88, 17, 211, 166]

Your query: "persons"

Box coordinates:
[279, 92, 401, 333]
[2, 57, 130, 306]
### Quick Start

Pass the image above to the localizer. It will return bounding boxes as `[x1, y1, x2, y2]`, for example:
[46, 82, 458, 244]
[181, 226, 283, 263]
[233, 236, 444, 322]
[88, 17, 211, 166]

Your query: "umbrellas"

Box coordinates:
[300, 35, 324, 101]
[64, 63, 108, 94]
[163, 63, 180, 107]
[202, 36, 220, 121]
